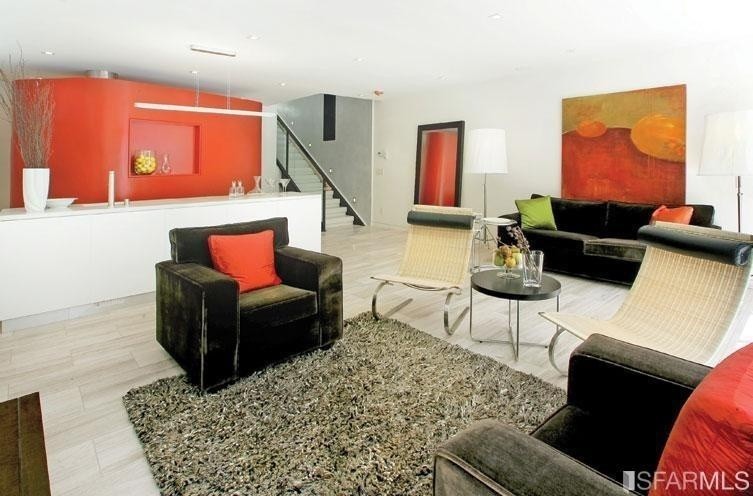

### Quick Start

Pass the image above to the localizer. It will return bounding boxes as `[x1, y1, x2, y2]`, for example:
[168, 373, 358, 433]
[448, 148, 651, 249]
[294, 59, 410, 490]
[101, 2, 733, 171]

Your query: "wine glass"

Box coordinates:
[493, 249, 522, 280]
[265, 177, 290, 196]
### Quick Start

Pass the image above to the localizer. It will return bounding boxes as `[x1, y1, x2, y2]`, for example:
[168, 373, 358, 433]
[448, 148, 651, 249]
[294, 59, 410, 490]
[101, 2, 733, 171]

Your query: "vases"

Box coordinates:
[21, 163, 51, 215]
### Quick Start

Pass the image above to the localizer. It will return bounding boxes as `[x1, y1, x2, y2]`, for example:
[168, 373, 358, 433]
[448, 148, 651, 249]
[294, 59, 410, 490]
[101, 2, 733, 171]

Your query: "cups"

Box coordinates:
[133, 148, 158, 175]
[521, 249, 543, 288]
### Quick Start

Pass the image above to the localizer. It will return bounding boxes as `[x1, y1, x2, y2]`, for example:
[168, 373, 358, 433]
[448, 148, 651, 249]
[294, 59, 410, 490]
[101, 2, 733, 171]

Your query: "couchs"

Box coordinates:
[495, 192, 724, 290]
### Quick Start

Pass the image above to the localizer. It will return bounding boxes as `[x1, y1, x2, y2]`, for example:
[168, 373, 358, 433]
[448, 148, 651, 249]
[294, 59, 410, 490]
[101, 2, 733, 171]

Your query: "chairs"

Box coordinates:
[534, 213, 752, 389]
[151, 212, 345, 390]
[367, 199, 480, 336]
[426, 330, 751, 495]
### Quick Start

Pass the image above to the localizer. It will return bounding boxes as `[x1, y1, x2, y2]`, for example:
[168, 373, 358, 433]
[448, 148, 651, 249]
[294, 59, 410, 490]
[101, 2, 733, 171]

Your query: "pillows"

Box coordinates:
[512, 194, 560, 235]
[207, 225, 283, 295]
[649, 202, 696, 226]
[644, 335, 751, 496]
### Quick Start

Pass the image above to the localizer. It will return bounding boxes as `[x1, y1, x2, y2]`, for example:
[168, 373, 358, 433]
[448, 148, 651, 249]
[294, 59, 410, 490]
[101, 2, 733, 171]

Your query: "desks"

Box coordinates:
[0, 190, 322, 329]
[471, 216, 519, 251]
[468, 268, 566, 362]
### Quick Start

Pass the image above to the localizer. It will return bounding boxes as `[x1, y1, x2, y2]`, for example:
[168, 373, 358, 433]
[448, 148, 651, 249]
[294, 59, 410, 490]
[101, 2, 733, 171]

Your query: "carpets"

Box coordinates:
[119, 305, 570, 496]
[0, 386, 51, 496]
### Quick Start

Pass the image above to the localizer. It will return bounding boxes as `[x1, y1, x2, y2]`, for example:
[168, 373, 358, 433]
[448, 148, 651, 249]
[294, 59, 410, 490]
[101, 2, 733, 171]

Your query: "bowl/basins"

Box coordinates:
[47, 198, 76, 210]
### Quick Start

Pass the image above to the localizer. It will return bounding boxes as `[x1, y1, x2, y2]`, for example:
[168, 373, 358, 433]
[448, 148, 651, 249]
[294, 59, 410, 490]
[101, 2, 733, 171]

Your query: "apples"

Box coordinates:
[494, 246, 521, 268]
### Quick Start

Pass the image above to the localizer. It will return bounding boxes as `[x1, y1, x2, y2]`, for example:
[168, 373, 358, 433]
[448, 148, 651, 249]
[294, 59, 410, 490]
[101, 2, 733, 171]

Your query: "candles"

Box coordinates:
[107, 169, 114, 207]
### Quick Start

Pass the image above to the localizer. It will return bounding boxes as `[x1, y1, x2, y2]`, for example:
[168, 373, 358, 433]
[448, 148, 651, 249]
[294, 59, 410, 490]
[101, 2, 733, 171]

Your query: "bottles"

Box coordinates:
[123, 198, 128, 205]
[228, 179, 237, 199]
[236, 180, 244, 197]
[247, 175, 264, 196]
[106, 170, 115, 208]
[161, 153, 172, 176]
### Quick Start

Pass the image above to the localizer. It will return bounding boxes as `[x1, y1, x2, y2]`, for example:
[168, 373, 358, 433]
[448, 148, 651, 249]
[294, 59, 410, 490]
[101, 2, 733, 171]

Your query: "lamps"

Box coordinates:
[131, 42, 280, 120]
[467, 125, 510, 247]
